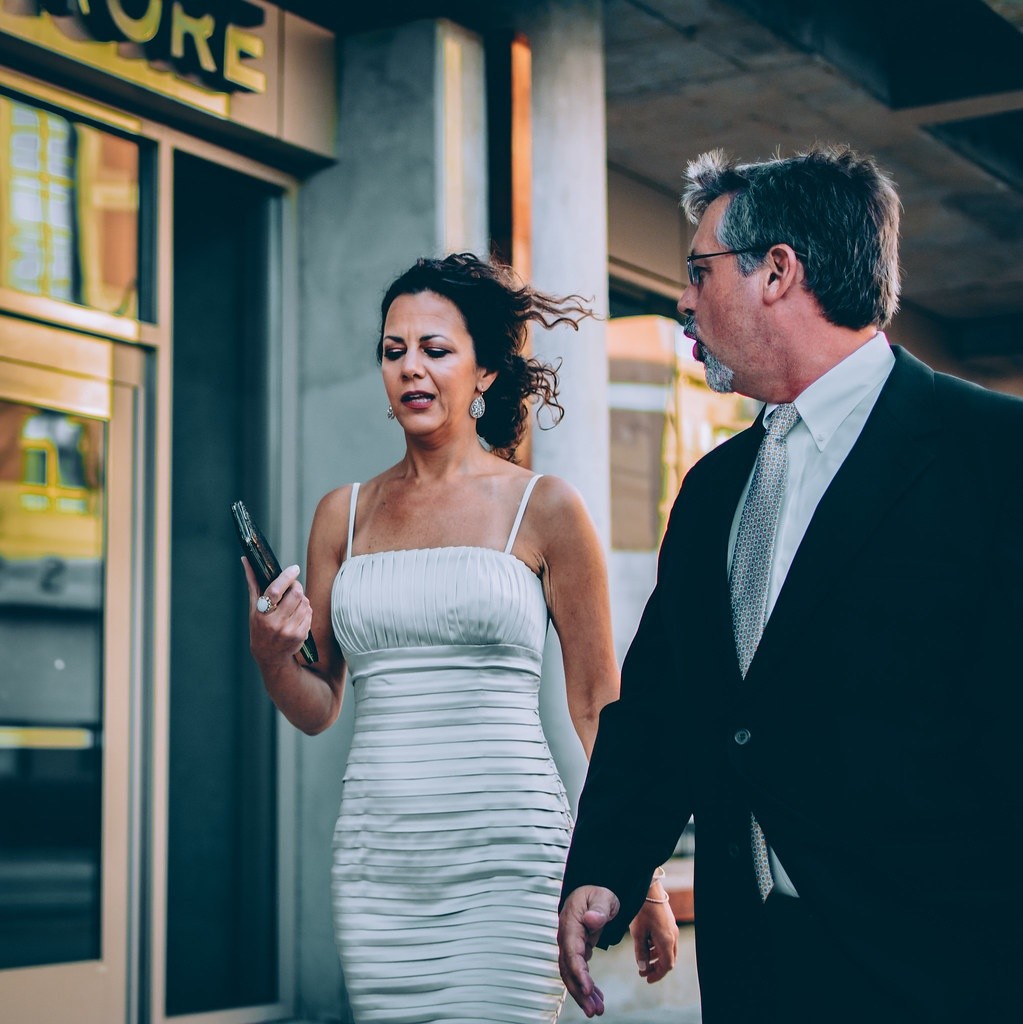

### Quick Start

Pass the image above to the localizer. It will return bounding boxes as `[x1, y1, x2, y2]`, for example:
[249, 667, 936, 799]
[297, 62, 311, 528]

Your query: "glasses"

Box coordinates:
[684, 244, 809, 289]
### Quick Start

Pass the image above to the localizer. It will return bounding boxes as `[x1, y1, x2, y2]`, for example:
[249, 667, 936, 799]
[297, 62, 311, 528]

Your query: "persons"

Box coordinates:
[242, 261, 678, 1024]
[558, 145, 1023, 1024]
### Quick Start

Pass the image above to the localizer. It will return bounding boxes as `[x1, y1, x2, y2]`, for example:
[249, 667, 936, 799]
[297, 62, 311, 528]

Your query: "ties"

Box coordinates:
[729, 400, 799, 904]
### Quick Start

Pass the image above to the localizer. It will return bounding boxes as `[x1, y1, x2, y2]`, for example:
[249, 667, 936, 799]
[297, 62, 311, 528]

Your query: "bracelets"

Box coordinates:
[645, 866, 669, 903]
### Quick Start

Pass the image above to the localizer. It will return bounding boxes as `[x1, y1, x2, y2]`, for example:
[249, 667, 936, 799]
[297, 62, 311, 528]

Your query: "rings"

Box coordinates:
[257, 596, 278, 614]
